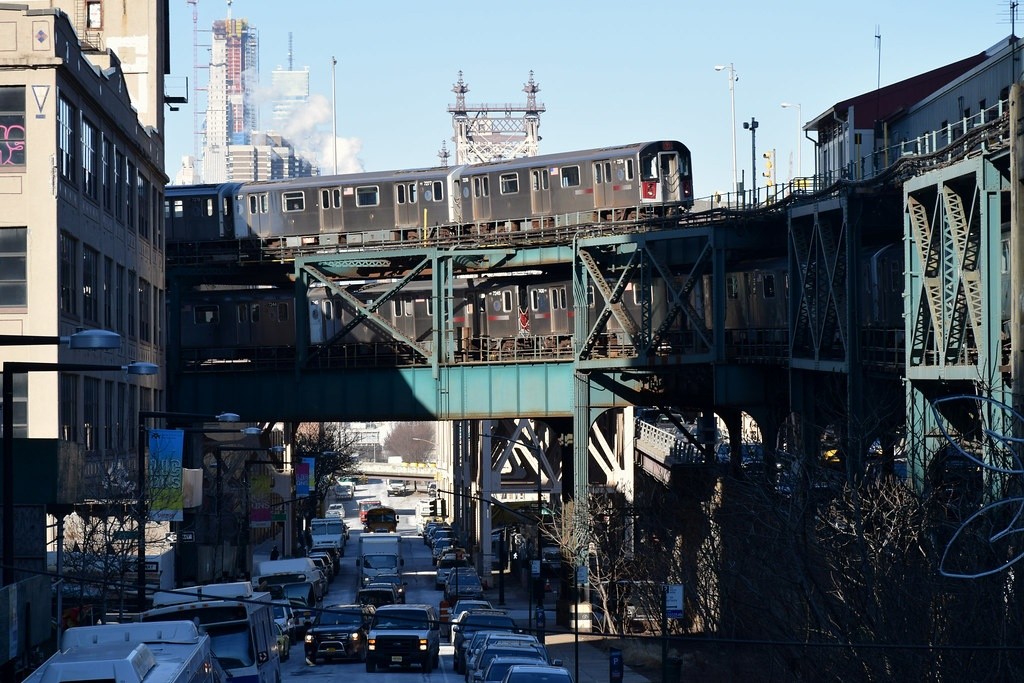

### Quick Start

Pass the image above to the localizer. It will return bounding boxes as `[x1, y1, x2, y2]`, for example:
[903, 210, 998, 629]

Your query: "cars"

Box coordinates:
[499, 666, 573, 683]
[655, 413, 701, 444]
[716, 440, 831, 504]
[258, 476, 548, 683]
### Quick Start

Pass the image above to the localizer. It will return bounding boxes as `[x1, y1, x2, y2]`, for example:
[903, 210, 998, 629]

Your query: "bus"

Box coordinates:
[137, 579, 283, 683]
[22, 621, 232, 682]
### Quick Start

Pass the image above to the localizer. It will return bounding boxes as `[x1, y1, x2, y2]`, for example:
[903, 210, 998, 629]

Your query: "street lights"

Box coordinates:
[133, 408, 245, 617]
[331, 55, 340, 174]
[1, 360, 161, 682]
[241, 451, 337, 575]
[742, 116, 760, 210]
[716, 61, 743, 210]
[213, 444, 287, 585]
[782, 102, 803, 185]
[49, 502, 76, 654]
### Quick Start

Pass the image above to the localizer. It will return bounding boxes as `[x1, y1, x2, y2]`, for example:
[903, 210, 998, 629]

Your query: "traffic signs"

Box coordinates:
[123, 561, 157, 573]
[164, 531, 195, 544]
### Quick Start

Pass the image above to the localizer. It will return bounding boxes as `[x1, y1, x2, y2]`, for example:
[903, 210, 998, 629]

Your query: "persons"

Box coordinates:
[294, 203, 300, 209]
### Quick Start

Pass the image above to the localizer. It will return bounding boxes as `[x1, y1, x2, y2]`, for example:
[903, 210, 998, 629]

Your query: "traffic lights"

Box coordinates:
[715, 193, 722, 202]
[763, 150, 773, 187]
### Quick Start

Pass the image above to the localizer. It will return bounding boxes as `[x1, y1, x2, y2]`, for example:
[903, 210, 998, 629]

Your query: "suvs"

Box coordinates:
[304, 604, 376, 666]
[474, 653, 550, 683]
[366, 605, 445, 670]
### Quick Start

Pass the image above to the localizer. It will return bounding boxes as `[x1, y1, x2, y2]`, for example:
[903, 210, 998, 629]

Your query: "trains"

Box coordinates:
[164, 139, 694, 259]
[169, 239, 904, 355]
